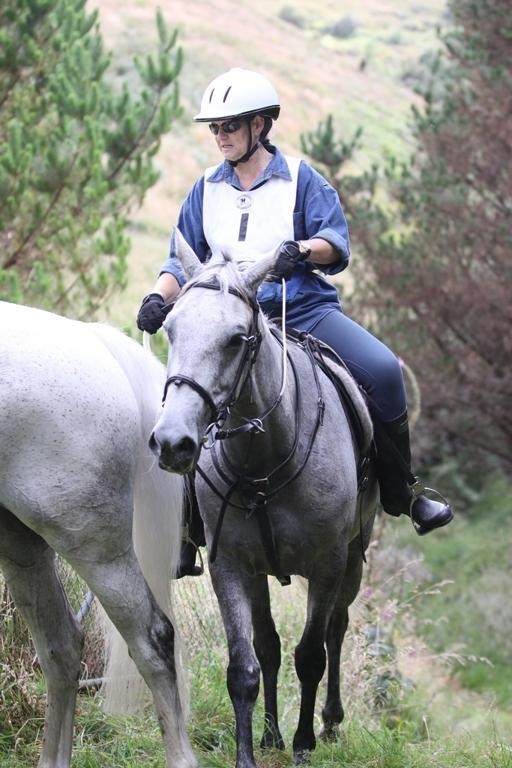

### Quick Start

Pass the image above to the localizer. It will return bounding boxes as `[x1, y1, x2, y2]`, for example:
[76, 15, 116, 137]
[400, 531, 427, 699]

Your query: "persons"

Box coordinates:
[135, 67, 455, 576]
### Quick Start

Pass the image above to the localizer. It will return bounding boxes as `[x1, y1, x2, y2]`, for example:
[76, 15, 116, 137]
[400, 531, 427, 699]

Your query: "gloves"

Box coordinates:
[136, 292, 169, 336]
[266, 238, 310, 286]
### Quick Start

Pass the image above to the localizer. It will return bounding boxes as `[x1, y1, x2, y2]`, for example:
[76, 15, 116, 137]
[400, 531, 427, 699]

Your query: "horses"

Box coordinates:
[144, 222, 421, 768]
[0, 299, 198, 768]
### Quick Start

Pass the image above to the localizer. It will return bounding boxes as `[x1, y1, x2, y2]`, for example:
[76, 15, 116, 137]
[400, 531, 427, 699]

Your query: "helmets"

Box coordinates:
[192, 65, 283, 122]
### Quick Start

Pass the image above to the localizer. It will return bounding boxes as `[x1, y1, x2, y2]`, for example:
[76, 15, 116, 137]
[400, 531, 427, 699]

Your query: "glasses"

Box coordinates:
[203, 114, 252, 134]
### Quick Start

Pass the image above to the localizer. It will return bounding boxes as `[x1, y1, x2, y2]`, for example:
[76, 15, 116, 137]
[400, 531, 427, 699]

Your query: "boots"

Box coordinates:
[375, 401, 451, 529]
[176, 469, 207, 578]
